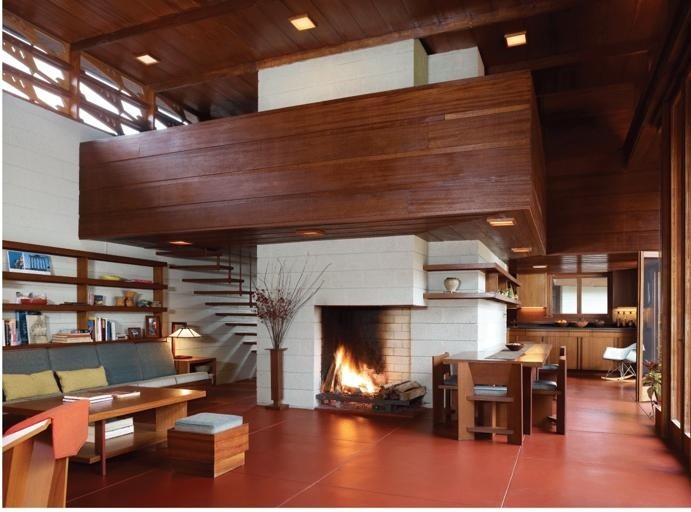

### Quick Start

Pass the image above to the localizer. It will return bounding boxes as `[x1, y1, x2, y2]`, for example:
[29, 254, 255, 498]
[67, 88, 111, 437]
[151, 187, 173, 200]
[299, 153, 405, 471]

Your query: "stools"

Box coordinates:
[165, 413, 249, 480]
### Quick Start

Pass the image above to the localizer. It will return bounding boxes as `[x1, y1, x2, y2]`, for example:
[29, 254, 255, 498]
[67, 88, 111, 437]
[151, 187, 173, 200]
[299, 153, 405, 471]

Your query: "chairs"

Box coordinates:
[600, 343, 646, 382]
[431, 346, 568, 445]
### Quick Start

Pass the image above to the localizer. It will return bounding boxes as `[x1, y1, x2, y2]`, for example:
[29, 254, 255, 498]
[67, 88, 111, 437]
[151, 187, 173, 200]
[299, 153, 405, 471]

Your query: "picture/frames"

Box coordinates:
[128, 328, 142, 338]
[173, 321, 192, 359]
[146, 315, 161, 338]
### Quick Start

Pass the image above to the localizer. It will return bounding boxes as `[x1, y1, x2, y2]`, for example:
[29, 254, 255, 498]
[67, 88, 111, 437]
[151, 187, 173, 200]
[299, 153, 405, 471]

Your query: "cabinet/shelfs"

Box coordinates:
[174, 357, 216, 389]
[582, 330, 635, 376]
[422, 263, 521, 309]
[510, 329, 577, 377]
[3, 240, 167, 343]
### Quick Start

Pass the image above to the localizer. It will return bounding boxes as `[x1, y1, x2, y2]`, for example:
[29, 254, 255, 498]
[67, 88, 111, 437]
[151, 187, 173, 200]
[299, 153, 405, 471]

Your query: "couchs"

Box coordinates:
[2, 339, 214, 424]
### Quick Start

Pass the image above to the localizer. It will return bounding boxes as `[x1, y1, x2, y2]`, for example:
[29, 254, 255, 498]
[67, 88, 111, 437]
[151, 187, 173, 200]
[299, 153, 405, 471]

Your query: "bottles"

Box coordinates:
[616, 311, 633, 327]
[444, 276, 459, 292]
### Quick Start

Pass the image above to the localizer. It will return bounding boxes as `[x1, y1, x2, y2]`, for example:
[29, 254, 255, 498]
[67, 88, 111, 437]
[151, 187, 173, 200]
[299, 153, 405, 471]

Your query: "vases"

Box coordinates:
[264, 346, 290, 411]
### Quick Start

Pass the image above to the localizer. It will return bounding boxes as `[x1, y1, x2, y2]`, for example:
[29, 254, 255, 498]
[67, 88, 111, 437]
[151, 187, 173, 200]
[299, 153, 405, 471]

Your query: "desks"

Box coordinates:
[487, 343, 554, 436]
[6, 384, 207, 477]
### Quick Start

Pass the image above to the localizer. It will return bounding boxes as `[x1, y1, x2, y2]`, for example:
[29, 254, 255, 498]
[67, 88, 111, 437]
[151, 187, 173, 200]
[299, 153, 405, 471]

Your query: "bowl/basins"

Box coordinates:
[505, 344, 524, 350]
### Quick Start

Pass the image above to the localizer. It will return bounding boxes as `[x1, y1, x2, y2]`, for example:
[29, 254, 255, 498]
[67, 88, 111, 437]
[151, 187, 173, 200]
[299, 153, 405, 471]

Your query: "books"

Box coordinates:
[51, 316, 116, 343]
[62, 391, 140, 404]
[2, 309, 49, 347]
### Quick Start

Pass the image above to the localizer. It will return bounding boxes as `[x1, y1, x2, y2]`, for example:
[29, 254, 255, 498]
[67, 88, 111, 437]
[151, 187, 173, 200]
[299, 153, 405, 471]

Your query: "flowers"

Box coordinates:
[251, 259, 323, 349]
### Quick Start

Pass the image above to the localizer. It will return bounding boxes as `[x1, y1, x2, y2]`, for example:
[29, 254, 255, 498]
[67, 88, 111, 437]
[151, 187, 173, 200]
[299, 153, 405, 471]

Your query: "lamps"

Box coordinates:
[169, 328, 201, 357]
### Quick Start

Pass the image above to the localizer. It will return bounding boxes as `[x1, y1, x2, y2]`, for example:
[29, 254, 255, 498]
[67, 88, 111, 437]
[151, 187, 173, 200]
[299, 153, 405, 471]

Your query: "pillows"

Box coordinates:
[2, 365, 108, 401]
